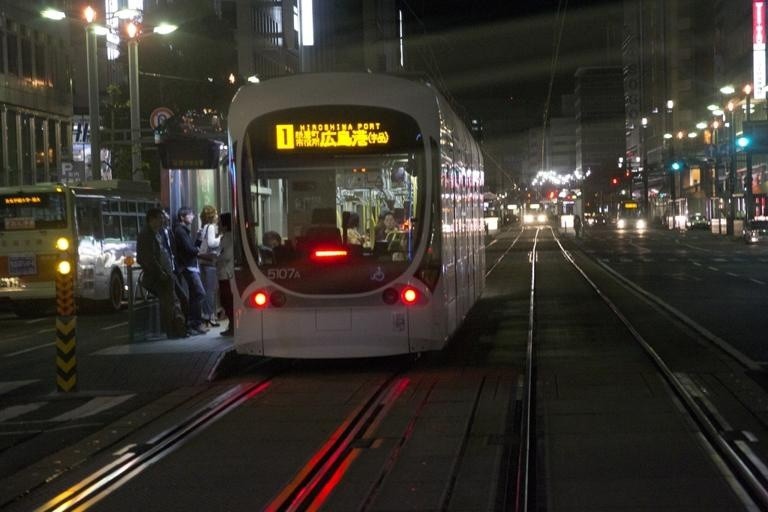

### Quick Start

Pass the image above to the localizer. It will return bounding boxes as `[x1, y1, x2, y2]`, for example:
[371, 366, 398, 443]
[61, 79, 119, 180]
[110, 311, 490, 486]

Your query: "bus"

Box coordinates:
[524, 202, 546, 226]
[0, 181, 170, 313]
[616, 201, 646, 229]
[227, 66, 486, 360]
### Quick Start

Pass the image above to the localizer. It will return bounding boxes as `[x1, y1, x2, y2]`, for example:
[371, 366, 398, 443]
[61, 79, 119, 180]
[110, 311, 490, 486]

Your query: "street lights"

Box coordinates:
[41, 5, 180, 183]
[663, 84, 768, 236]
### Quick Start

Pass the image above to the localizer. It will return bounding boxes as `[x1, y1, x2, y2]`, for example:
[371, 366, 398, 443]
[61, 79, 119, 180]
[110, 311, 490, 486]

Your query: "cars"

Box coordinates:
[592, 213, 608, 225]
[744, 216, 768, 243]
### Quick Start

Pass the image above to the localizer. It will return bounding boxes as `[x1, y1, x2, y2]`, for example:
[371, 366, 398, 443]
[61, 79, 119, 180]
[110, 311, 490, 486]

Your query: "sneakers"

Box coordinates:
[170, 320, 234, 337]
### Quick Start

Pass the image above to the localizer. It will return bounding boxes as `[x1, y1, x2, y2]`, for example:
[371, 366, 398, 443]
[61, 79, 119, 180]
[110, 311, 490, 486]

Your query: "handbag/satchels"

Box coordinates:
[197, 224, 222, 262]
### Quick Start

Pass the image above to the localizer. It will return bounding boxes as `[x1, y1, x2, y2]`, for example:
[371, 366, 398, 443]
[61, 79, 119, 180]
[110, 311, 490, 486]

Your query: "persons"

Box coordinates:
[343, 201, 402, 245]
[94, 215, 119, 235]
[573, 214, 582, 237]
[485, 223, 489, 236]
[136, 205, 281, 337]
